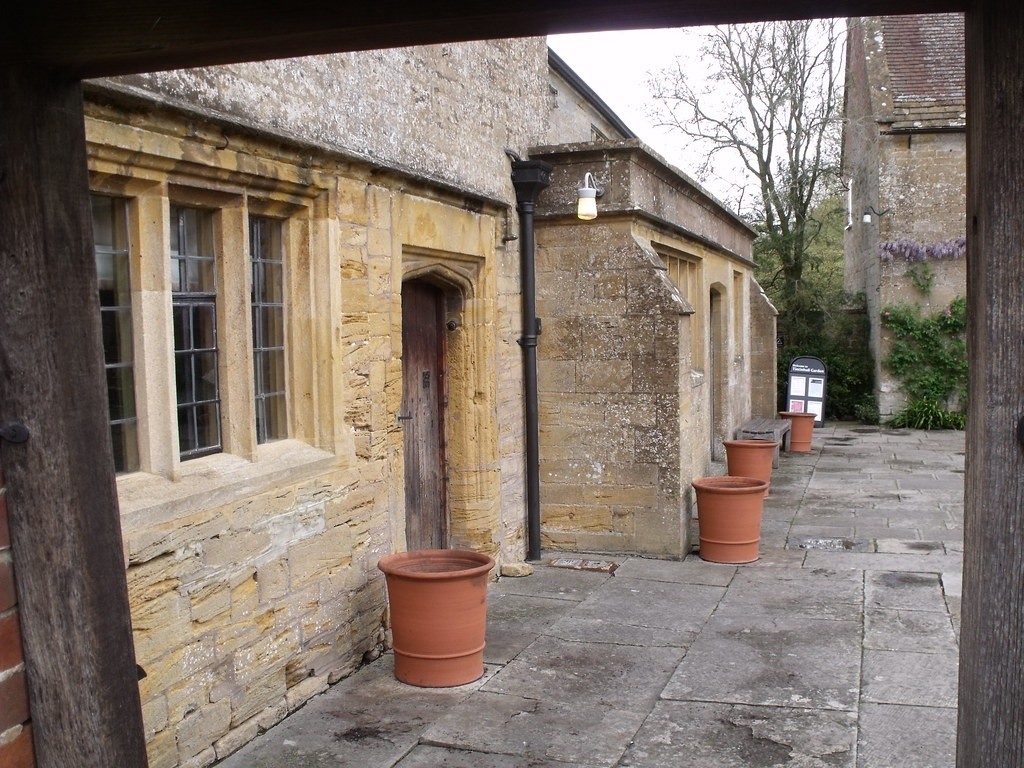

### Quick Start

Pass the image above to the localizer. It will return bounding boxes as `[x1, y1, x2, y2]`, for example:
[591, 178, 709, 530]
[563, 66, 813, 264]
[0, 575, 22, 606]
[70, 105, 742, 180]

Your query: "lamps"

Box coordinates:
[577, 171, 605, 221]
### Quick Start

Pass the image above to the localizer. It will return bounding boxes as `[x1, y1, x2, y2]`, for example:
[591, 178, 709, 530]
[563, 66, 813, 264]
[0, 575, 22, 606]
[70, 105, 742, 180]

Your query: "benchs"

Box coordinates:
[735, 417, 792, 468]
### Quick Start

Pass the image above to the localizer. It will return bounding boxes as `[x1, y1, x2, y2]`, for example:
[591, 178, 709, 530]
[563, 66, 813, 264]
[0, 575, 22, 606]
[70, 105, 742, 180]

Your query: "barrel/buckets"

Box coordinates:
[722, 439, 778, 496]
[779, 411, 818, 452]
[691, 475, 771, 564]
[377, 549, 497, 689]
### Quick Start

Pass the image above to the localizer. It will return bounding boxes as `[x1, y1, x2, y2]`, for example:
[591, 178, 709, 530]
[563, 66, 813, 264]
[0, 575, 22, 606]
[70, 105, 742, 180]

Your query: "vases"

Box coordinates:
[691, 476, 767, 564]
[778, 411, 817, 452]
[378, 549, 495, 688]
[723, 439, 779, 497]
